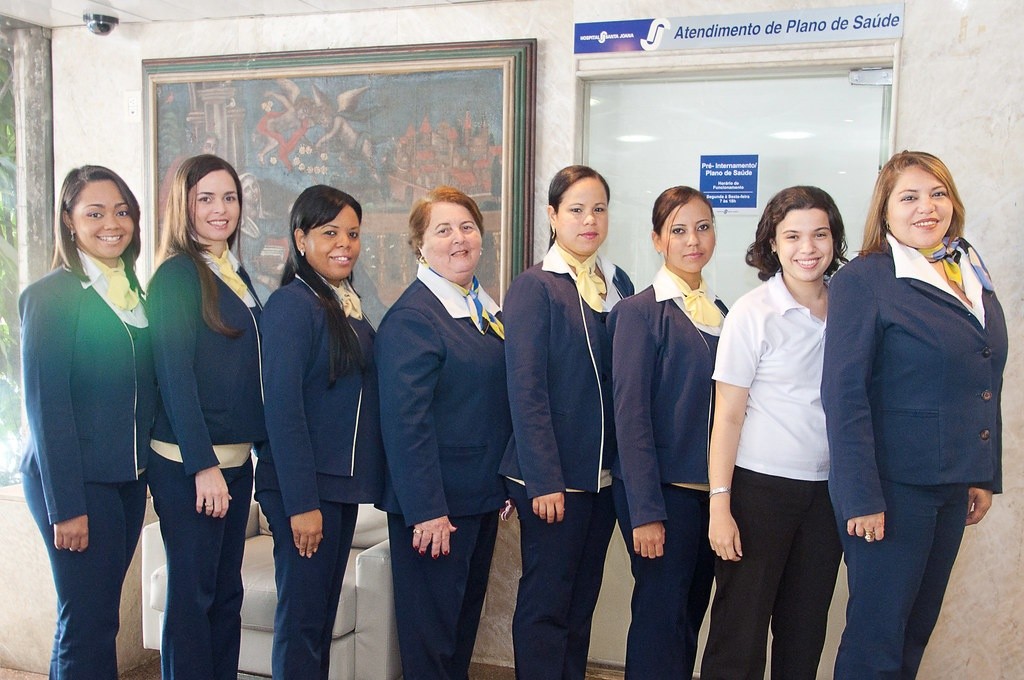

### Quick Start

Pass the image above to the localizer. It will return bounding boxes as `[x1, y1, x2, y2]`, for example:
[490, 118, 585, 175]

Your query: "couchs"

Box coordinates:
[140, 448, 403, 680]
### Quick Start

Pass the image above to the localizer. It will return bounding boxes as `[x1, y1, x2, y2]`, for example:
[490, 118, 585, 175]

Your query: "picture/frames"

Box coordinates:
[141, 38, 539, 332]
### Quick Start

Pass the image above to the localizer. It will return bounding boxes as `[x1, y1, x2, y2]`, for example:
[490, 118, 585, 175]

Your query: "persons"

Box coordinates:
[377, 189, 515, 680]
[501, 163, 635, 680]
[138, 151, 266, 680]
[814, 149, 1010, 680]
[705, 184, 844, 680]
[18, 163, 154, 680]
[259, 184, 380, 680]
[605, 185, 738, 680]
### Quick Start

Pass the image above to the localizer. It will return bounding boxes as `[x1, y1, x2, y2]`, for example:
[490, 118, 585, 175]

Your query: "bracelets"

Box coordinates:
[709, 487, 731, 497]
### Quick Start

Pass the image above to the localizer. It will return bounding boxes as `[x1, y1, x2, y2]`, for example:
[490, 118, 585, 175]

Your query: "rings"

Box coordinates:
[205, 503, 212, 507]
[413, 528, 422, 533]
[864, 531, 874, 542]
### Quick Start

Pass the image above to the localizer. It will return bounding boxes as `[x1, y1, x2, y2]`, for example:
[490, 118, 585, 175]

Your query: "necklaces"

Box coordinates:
[928, 259, 940, 263]
